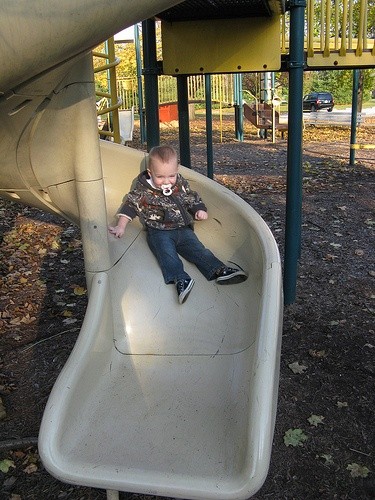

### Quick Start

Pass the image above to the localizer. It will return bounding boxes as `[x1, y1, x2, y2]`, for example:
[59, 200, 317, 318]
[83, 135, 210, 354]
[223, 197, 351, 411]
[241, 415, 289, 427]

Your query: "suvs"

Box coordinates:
[303, 92, 335, 112]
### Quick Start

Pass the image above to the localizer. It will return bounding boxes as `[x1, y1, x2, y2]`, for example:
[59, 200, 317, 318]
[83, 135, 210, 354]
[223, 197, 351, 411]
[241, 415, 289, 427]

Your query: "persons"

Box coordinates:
[108, 144, 249, 305]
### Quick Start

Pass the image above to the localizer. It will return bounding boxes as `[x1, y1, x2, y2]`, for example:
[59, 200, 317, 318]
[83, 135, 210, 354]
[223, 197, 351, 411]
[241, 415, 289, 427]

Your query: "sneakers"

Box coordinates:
[216, 267, 248, 285]
[176, 279, 195, 304]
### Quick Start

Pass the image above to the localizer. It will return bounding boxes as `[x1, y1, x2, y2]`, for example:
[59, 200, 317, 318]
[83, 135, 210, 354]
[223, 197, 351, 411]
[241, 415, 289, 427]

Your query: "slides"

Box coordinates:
[0, 0, 285, 500]
